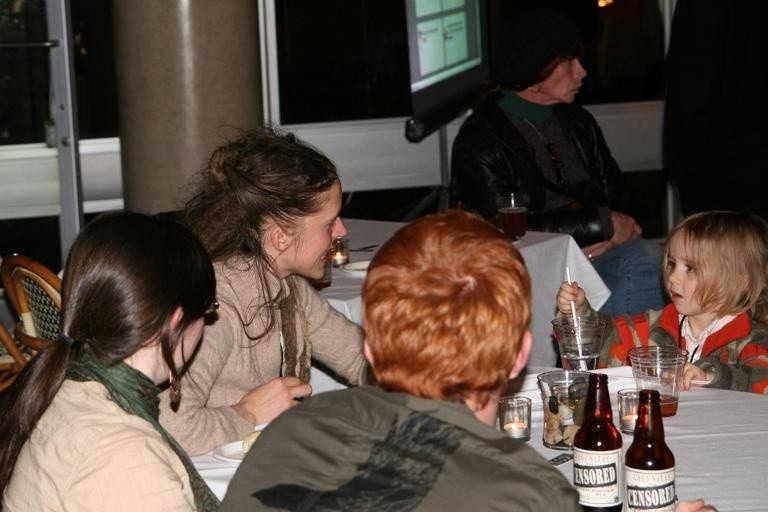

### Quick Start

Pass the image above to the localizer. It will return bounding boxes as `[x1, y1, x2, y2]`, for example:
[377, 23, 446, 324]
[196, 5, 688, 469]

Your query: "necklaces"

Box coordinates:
[675, 314, 700, 363]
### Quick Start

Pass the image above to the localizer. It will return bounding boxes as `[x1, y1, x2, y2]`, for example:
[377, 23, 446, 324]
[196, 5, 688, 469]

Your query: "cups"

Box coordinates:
[627, 345, 688, 417]
[552, 316, 612, 372]
[537, 370, 589, 450]
[496, 193, 531, 237]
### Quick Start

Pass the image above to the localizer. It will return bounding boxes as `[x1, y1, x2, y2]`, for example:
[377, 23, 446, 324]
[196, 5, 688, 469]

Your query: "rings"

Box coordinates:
[588, 253, 592, 259]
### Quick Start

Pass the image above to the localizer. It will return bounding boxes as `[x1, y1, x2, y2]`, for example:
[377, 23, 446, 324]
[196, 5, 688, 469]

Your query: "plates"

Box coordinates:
[340, 259, 371, 273]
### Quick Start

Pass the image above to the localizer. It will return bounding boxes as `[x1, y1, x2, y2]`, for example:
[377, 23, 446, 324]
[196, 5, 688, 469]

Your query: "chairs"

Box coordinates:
[0, 252, 65, 361]
[396, 87, 487, 224]
[0, 321, 33, 395]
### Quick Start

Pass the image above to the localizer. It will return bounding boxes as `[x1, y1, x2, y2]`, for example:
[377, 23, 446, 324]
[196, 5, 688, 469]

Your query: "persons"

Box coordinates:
[451, 22, 664, 318]
[157, 126, 370, 458]
[218, 209, 584, 512]
[1, 213, 219, 512]
[551, 210, 768, 393]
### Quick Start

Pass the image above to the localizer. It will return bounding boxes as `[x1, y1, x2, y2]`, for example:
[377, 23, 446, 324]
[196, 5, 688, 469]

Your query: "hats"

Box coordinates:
[493, 28, 581, 93]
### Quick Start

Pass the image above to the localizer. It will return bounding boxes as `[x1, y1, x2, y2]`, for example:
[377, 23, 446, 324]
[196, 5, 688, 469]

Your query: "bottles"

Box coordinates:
[497, 397, 531, 441]
[573, 374, 624, 511]
[625, 390, 676, 512]
[334, 238, 349, 268]
[618, 388, 641, 434]
